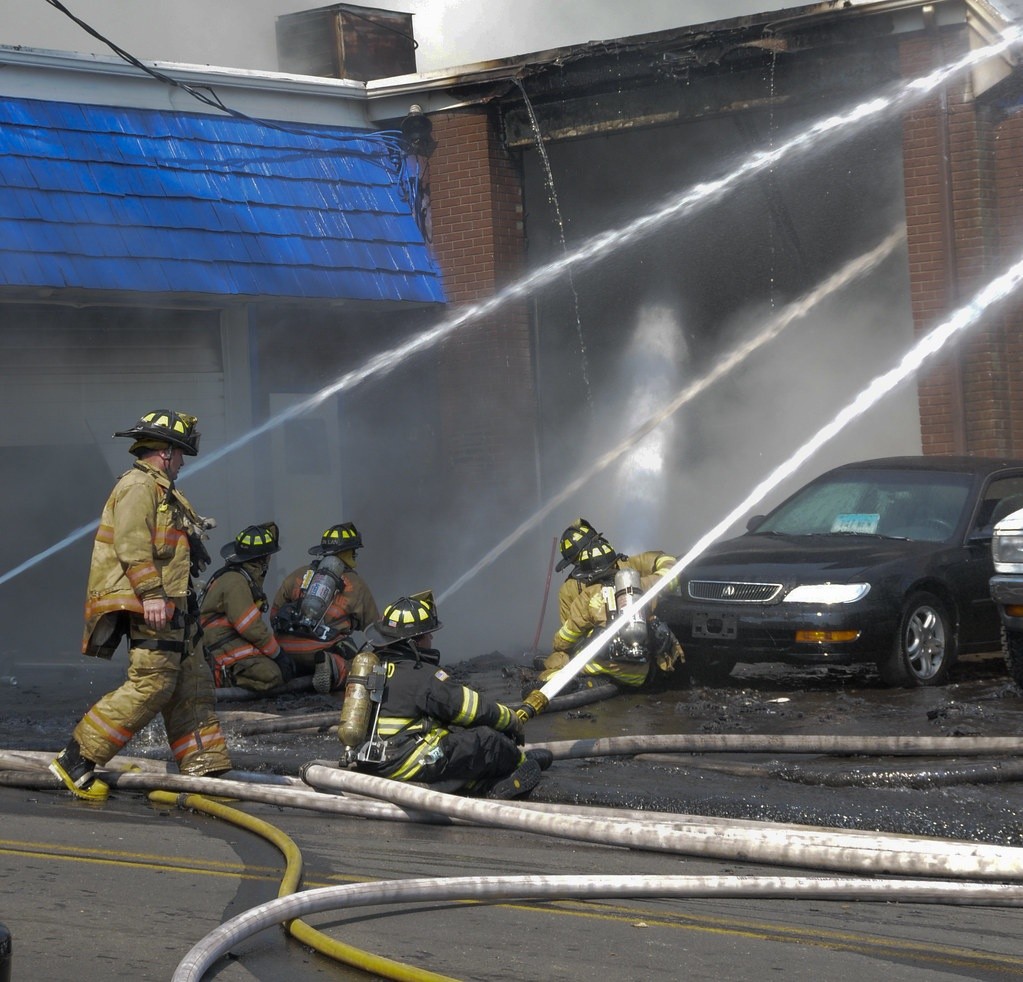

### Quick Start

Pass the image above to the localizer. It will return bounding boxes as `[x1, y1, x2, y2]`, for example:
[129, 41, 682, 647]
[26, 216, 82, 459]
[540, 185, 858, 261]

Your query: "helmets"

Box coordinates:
[221, 523, 281, 563]
[570, 538, 618, 578]
[364, 590, 444, 647]
[308, 522, 365, 555]
[114, 410, 200, 456]
[554, 518, 598, 572]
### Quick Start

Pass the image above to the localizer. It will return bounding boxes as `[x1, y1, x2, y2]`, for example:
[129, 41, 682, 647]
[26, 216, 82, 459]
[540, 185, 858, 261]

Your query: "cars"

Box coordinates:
[657, 455, 1022, 689]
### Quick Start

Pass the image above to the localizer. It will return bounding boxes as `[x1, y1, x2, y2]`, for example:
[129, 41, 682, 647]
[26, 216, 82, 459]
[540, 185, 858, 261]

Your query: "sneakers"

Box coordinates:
[523, 748, 553, 771]
[491, 760, 542, 800]
[48, 739, 110, 800]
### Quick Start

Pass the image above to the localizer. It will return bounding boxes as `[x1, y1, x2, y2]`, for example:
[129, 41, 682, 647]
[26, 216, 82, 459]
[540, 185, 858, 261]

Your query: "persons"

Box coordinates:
[270, 521, 381, 693]
[353, 596, 554, 800]
[49, 410, 231, 800]
[197, 521, 284, 692]
[534, 517, 665, 670]
[520, 542, 682, 699]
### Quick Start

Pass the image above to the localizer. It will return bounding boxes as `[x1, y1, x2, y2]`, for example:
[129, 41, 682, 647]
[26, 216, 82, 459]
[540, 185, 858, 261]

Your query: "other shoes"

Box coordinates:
[520, 680, 580, 701]
[312, 651, 333, 692]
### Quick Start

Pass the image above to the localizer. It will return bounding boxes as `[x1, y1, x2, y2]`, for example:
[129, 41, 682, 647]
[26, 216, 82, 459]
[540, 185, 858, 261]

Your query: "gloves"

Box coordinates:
[187, 534, 211, 578]
[502, 709, 525, 747]
[273, 647, 293, 680]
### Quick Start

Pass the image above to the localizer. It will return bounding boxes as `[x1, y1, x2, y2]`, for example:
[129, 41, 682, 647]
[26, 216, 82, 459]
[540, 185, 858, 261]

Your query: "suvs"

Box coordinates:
[991, 506, 1023, 689]
